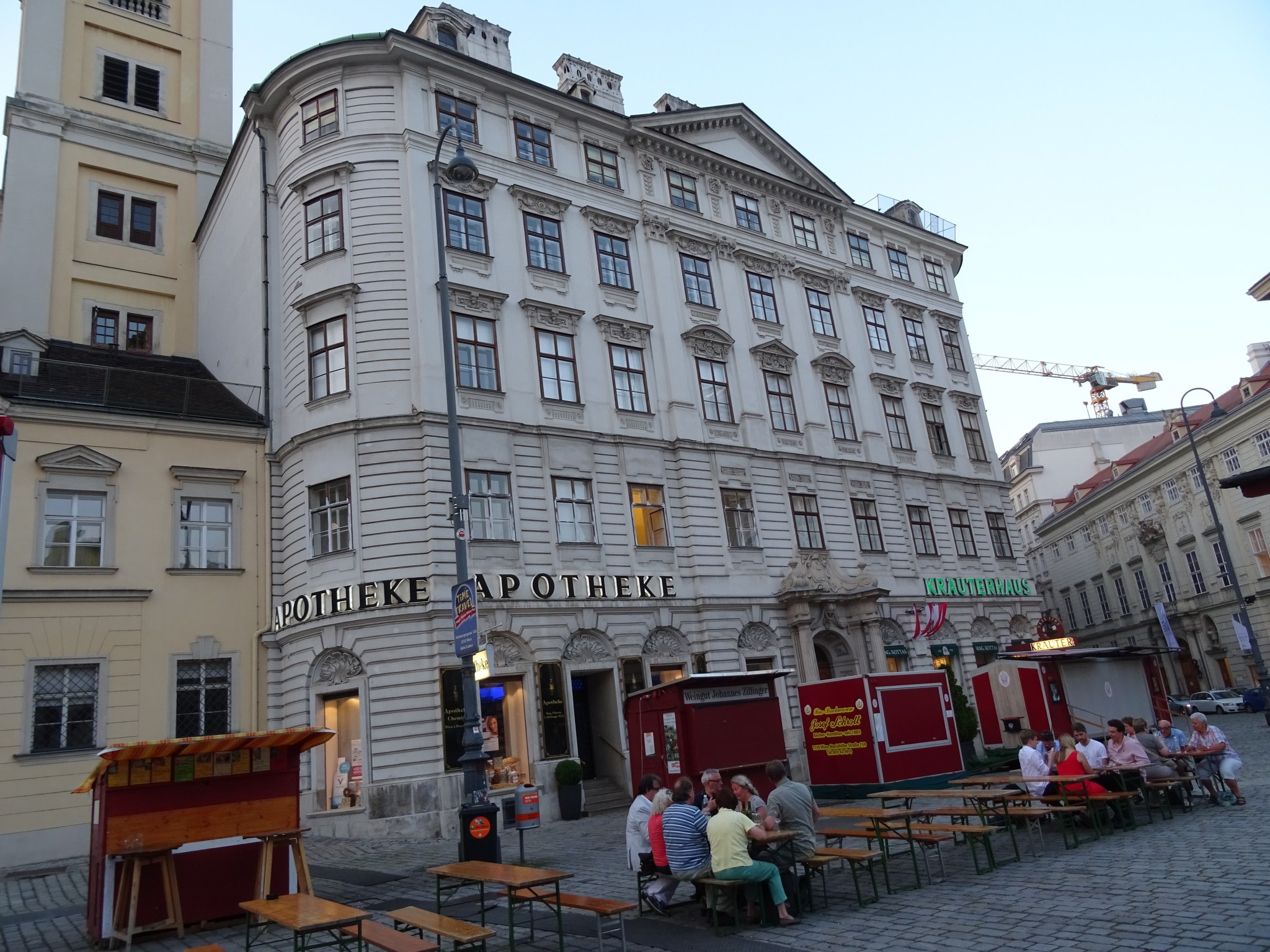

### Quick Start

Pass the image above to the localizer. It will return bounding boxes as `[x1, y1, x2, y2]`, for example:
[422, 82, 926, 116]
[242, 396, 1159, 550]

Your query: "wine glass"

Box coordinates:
[773, 810, 782, 833]
[1186, 737, 1194, 752]
[1179, 739, 1187, 753]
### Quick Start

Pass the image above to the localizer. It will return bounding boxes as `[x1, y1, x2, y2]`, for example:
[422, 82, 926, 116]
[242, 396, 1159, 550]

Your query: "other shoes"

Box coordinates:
[690, 892, 697, 899]
[705, 910, 714, 924]
[696, 897, 707, 905]
[746, 913, 760, 923]
[1110, 812, 1120, 822]
[1132, 795, 1143, 804]
[1079, 811, 1093, 829]
[742, 902, 747, 910]
[779, 916, 800, 927]
[718, 912, 735, 926]
[799, 877, 809, 910]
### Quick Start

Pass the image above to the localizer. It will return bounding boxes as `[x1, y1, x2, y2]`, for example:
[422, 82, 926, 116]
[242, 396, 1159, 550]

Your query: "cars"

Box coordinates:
[1242, 686, 1266, 714]
[1166, 694, 1191, 716]
[1188, 690, 1246, 714]
[1215, 687, 1248, 695]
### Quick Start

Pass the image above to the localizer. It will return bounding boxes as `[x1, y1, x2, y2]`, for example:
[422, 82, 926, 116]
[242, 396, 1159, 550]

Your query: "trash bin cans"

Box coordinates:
[515, 783, 541, 831]
[457, 800, 501, 862]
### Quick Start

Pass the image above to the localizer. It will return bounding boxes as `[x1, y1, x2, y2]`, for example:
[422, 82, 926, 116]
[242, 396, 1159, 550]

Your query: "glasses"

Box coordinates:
[666, 789, 672, 794]
[1158, 726, 1170, 730]
[652, 788, 660, 791]
[692, 788, 696, 792]
[710, 777, 722, 783]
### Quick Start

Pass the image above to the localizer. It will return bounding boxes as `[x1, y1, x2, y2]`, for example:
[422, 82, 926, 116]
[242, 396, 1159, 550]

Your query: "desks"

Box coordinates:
[748, 829, 805, 923]
[426, 860, 574, 952]
[1168, 749, 1225, 792]
[817, 807, 922, 894]
[1094, 763, 1156, 829]
[867, 790, 1022, 866]
[948, 775, 1099, 849]
[238, 892, 373, 952]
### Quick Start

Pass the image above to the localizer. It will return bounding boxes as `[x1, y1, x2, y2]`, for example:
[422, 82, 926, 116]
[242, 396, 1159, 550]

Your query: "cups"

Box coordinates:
[1084, 750, 1090, 760]
[1195, 744, 1201, 751]
[753, 813, 762, 825]
[1151, 728, 1158, 737]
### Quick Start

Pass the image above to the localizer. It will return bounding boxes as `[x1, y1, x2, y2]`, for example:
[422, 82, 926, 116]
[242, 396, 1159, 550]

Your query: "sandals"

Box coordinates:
[1237, 797, 1245, 804]
[1208, 796, 1213, 804]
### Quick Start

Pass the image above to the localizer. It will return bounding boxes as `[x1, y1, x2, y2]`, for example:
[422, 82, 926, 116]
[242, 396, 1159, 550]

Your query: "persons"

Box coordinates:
[485, 715, 498, 737]
[625, 759, 821, 927]
[1017, 712, 1246, 832]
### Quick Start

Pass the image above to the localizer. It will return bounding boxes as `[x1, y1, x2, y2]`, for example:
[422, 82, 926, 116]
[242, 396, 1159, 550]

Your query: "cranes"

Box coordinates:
[969, 353, 1162, 415]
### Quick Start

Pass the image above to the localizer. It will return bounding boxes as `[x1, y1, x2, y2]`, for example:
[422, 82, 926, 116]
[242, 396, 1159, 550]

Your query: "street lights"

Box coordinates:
[426, 123, 506, 864]
[1179, 386, 1270, 727]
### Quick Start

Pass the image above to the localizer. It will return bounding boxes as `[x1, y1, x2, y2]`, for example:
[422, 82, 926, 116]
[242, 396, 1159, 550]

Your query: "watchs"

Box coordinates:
[1207, 746, 1210, 750]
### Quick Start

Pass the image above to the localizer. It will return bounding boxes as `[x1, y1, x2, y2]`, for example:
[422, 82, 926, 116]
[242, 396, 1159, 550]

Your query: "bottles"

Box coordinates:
[1174, 737, 1181, 754]
[747, 801, 755, 821]
[703, 808, 711, 821]
[741, 801, 748, 817]
[702, 795, 709, 812]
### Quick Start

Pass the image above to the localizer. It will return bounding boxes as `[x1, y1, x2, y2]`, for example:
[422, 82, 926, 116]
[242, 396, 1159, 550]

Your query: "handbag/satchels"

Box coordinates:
[1001, 784, 1026, 794]
[638, 852, 656, 871]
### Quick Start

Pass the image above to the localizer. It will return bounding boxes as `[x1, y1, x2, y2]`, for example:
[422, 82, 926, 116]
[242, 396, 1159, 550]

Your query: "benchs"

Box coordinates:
[816, 766, 1242, 884]
[796, 856, 840, 911]
[817, 848, 883, 908]
[637, 873, 742, 936]
[499, 889, 638, 952]
[338, 906, 496, 952]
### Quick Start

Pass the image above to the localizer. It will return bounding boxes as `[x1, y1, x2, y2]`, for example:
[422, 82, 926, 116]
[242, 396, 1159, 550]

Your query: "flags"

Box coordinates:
[911, 602, 948, 639]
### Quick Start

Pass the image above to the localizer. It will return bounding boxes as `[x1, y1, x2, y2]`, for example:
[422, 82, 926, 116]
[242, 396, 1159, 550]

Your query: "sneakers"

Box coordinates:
[641, 891, 648, 901]
[645, 896, 669, 916]
